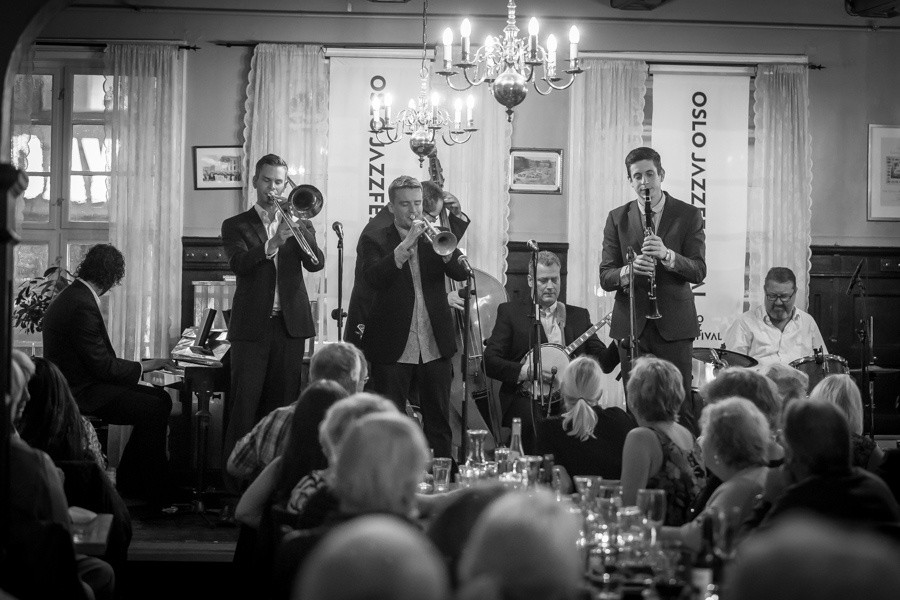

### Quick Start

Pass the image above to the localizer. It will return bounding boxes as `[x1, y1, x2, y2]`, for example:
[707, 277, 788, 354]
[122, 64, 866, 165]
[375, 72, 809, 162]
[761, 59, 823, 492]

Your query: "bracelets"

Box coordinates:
[662, 247, 670, 262]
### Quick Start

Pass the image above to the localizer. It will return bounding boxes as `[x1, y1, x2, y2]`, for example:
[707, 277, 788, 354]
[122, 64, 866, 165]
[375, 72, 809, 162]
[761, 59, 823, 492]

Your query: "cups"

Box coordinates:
[428, 429, 667, 599]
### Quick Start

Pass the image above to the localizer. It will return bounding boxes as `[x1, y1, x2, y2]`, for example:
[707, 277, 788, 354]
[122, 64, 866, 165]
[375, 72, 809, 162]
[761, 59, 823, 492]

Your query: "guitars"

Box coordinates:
[517, 309, 613, 403]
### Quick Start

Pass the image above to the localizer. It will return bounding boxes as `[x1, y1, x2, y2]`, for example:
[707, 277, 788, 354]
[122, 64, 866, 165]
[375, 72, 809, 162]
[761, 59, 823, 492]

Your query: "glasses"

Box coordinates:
[766, 290, 795, 302]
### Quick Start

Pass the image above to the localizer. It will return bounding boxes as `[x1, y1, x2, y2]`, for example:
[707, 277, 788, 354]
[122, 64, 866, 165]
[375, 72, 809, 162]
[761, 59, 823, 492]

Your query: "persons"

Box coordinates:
[227, 341, 900, 600]
[221, 154, 325, 455]
[599, 147, 707, 414]
[713, 267, 829, 378]
[343, 175, 471, 482]
[41, 243, 175, 500]
[481, 251, 621, 436]
[0, 349, 133, 600]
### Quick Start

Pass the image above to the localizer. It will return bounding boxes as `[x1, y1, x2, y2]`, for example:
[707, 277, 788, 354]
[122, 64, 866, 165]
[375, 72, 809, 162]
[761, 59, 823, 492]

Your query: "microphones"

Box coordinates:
[527, 240, 539, 252]
[332, 222, 344, 240]
[457, 255, 475, 278]
[551, 366, 558, 375]
[626, 246, 637, 262]
[847, 259, 864, 295]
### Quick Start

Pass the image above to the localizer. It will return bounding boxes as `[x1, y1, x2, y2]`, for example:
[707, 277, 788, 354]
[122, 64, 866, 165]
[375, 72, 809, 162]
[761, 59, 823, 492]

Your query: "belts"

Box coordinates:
[271, 311, 281, 316]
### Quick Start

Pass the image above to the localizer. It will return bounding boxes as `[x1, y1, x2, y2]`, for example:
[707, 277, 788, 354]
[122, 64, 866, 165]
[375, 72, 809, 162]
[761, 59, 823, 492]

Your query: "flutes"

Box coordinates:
[643, 188, 663, 322]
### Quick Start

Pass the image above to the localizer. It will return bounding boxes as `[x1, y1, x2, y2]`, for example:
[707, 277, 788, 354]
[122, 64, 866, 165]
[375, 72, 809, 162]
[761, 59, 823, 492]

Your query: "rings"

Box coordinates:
[545, 379, 547, 381]
[637, 265, 640, 269]
[458, 300, 461, 305]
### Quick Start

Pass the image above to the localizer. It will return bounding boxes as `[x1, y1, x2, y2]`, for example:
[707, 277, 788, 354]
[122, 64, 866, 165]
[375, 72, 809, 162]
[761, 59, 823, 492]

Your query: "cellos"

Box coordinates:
[428, 150, 514, 452]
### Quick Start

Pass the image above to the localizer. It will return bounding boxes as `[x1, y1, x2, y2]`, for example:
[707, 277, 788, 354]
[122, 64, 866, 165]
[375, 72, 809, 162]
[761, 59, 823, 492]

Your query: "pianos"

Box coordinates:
[135, 354, 231, 512]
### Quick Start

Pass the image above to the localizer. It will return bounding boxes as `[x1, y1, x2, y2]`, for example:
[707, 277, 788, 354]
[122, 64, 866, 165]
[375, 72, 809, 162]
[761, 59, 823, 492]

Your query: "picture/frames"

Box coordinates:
[192, 144, 248, 190]
[508, 147, 565, 195]
[867, 124, 900, 222]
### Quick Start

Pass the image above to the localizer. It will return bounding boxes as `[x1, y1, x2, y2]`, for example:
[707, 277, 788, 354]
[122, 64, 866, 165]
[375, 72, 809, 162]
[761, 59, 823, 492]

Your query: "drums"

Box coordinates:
[787, 354, 851, 395]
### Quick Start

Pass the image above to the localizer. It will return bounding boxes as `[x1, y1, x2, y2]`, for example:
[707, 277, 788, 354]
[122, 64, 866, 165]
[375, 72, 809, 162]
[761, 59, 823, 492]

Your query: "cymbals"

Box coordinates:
[851, 366, 900, 380]
[693, 346, 759, 369]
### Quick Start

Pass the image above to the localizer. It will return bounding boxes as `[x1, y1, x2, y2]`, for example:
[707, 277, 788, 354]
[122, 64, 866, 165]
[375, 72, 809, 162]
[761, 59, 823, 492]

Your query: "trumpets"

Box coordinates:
[408, 212, 458, 257]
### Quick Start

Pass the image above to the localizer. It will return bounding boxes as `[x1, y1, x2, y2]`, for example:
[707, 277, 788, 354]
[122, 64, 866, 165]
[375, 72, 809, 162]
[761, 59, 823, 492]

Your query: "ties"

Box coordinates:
[650, 210, 656, 235]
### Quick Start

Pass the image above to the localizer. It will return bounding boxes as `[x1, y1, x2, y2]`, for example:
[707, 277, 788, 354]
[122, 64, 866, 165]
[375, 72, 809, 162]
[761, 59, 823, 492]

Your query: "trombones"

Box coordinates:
[268, 174, 325, 265]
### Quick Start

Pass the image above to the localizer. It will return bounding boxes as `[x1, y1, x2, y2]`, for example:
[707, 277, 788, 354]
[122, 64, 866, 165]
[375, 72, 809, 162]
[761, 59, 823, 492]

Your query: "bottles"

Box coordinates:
[509, 417, 524, 457]
[466, 430, 488, 467]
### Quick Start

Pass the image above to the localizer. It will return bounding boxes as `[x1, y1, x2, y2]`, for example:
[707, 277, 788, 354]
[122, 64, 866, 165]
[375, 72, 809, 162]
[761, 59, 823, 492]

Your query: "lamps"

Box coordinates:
[370, 0, 479, 170]
[434, 0, 591, 125]
